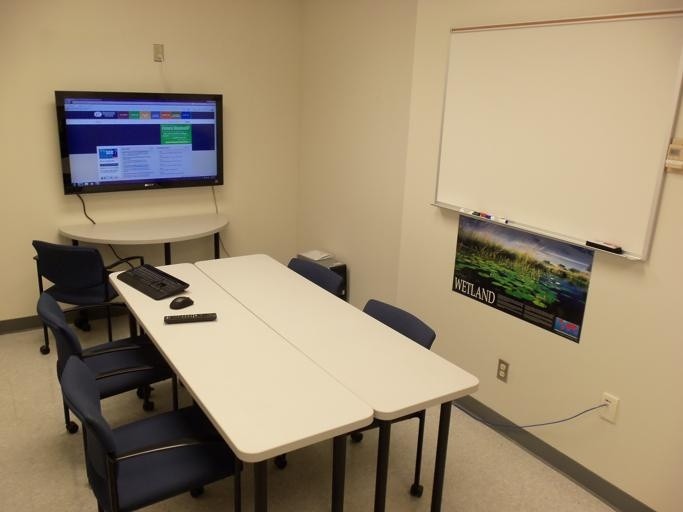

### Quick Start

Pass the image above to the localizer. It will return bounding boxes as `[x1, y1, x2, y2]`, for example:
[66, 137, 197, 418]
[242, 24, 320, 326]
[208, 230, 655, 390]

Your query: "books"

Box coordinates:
[299, 250, 331, 261]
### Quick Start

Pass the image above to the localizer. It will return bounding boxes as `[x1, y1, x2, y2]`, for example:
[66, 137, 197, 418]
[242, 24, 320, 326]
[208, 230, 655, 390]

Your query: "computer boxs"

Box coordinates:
[298, 254, 348, 300]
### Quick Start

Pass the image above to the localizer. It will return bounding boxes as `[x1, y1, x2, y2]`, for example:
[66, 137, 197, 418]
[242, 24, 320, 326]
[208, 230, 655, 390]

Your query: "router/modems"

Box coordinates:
[306, 250, 334, 260]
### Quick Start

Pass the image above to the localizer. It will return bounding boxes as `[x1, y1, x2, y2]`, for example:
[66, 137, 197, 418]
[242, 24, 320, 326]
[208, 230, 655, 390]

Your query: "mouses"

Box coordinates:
[170, 296, 194, 309]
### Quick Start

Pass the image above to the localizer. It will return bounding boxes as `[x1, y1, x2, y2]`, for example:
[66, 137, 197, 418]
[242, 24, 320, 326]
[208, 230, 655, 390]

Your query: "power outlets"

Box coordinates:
[597, 390, 621, 425]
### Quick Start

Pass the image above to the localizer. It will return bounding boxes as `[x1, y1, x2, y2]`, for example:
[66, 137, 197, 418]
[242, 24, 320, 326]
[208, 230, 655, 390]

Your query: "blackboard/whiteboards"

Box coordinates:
[429, 9, 683, 262]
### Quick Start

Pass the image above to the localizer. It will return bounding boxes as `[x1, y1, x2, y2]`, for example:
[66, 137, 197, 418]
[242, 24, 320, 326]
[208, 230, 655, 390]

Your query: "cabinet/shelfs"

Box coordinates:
[34, 285, 179, 434]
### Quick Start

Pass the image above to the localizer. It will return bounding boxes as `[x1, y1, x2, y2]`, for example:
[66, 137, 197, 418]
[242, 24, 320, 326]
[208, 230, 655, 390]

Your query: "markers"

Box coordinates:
[459, 208, 509, 224]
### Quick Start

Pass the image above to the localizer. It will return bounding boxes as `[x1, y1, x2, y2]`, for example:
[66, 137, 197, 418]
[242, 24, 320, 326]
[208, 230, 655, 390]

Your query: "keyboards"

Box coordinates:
[117, 264, 190, 301]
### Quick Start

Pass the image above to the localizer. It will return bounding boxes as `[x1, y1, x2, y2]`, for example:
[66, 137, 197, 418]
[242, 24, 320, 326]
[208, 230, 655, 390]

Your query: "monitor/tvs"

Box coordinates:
[55, 91, 223, 196]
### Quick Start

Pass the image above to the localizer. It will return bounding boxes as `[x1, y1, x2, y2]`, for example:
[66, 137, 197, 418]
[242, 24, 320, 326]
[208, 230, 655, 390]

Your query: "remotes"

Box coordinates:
[165, 312, 217, 324]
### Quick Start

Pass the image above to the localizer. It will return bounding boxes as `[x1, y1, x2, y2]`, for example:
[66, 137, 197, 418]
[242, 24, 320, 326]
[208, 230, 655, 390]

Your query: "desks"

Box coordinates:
[194, 254, 480, 511]
[106, 262, 373, 511]
[61, 214, 230, 265]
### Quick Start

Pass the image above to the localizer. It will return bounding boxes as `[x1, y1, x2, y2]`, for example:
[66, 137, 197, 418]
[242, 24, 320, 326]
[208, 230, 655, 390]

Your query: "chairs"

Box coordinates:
[57, 354, 247, 512]
[352, 294, 435, 497]
[29, 238, 153, 356]
[281, 254, 342, 296]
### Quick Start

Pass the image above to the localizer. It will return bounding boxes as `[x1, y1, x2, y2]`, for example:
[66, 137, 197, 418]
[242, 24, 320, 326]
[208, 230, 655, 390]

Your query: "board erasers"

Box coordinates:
[586, 239, 622, 254]
